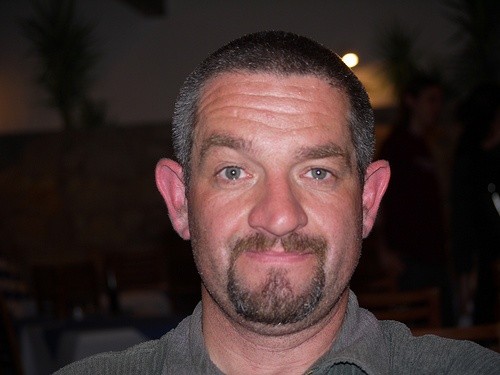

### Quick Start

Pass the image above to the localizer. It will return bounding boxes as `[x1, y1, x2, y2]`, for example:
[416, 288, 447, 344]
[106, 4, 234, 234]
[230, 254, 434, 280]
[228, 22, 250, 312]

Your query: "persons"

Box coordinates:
[360, 51, 500, 350]
[53, 30, 500, 374]
